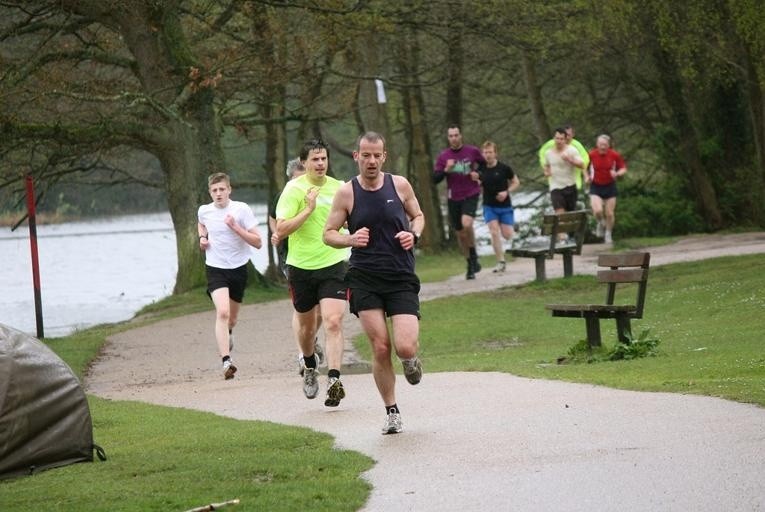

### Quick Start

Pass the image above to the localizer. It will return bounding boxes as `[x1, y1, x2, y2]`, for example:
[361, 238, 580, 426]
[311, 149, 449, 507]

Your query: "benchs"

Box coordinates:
[543, 251, 651, 347]
[505, 208, 591, 281]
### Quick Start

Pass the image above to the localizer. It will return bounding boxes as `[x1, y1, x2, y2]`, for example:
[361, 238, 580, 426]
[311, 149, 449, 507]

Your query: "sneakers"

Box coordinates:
[222, 358, 236, 383]
[297, 353, 321, 400]
[595, 223, 605, 237]
[492, 261, 506, 273]
[379, 408, 405, 434]
[473, 257, 482, 274]
[228, 329, 235, 354]
[400, 356, 425, 386]
[603, 237, 614, 252]
[311, 337, 325, 366]
[323, 377, 346, 409]
[467, 264, 476, 280]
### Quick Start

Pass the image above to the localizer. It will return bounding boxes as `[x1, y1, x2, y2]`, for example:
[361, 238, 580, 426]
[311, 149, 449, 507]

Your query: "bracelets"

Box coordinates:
[198, 235, 207, 239]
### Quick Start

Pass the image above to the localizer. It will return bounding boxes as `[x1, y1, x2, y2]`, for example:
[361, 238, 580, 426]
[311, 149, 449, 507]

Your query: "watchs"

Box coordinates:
[408, 230, 418, 244]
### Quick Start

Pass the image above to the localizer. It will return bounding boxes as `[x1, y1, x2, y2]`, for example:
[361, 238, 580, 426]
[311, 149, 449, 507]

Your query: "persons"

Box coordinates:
[275, 138, 352, 409]
[537, 124, 590, 188]
[480, 141, 519, 276]
[323, 132, 427, 435]
[543, 128, 585, 245]
[583, 134, 626, 243]
[430, 124, 487, 281]
[267, 156, 324, 374]
[197, 173, 263, 379]
